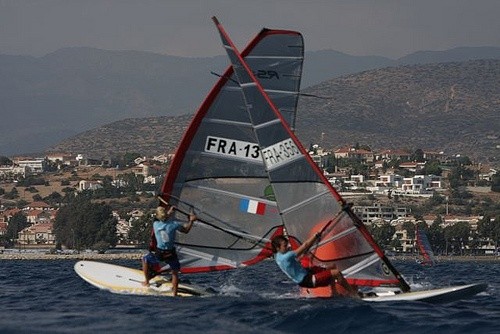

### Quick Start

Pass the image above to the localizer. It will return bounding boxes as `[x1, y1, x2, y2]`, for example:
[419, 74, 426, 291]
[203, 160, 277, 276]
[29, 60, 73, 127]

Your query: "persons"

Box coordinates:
[141, 206, 197, 297]
[271, 233, 363, 298]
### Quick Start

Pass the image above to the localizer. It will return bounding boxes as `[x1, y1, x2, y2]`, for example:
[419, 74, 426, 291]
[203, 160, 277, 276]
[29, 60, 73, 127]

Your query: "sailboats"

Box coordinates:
[416, 226, 439, 265]
[74, 25, 304, 298]
[211, 15, 488, 306]
